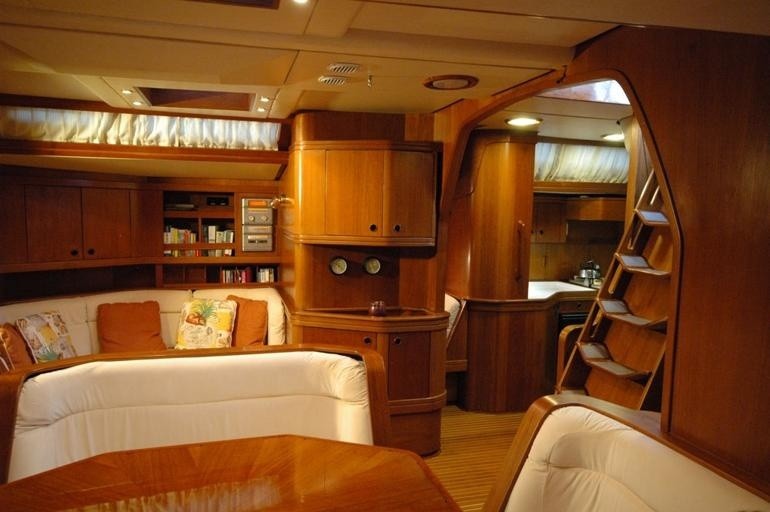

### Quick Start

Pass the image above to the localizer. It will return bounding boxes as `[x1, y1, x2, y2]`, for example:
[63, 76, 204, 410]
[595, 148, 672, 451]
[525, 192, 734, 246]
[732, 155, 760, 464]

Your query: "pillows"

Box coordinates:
[0, 295, 267, 372]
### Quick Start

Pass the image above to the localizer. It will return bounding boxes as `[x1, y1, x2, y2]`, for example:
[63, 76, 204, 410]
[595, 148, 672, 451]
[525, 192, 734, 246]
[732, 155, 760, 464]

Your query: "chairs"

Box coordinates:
[480, 394, 770, 512]
[0, 342, 391, 484]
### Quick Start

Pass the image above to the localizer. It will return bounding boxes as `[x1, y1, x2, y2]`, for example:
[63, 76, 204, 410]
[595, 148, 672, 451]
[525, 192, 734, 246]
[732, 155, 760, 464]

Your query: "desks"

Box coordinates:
[0, 435, 461, 512]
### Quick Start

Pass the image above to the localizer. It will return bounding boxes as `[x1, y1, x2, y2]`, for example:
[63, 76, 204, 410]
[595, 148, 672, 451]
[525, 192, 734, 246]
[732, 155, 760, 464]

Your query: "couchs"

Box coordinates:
[0, 283, 286, 373]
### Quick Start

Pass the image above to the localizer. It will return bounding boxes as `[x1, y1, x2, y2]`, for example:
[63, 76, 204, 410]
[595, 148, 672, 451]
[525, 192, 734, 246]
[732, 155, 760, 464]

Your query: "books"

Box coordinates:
[160, 198, 280, 285]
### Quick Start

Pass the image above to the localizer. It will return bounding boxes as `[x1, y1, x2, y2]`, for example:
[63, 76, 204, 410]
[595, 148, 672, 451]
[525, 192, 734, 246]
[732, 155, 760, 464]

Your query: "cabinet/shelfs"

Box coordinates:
[302, 326, 430, 403]
[0, 166, 235, 275]
[325, 148, 436, 240]
[531, 199, 567, 244]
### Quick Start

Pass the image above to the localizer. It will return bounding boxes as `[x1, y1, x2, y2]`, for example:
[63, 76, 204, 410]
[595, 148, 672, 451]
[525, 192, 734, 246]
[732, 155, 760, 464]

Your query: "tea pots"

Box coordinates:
[579, 259, 601, 279]
